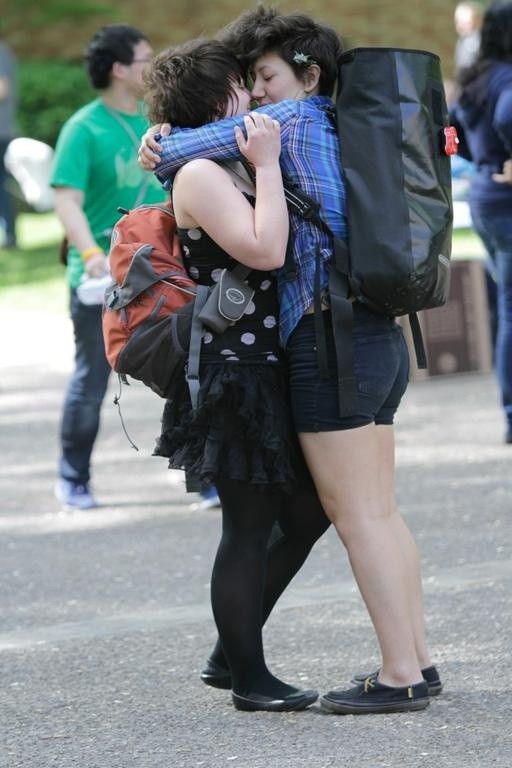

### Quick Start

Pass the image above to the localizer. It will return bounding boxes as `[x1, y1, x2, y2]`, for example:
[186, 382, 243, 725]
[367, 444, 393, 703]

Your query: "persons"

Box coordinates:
[136, 37, 334, 711]
[137, 3, 445, 714]
[453, 3, 482, 71]
[47, 25, 223, 511]
[0, 39, 24, 246]
[448, 1, 512, 444]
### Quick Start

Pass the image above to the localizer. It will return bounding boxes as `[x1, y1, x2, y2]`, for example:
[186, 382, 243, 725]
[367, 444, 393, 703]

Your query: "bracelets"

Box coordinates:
[80, 246, 104, 263]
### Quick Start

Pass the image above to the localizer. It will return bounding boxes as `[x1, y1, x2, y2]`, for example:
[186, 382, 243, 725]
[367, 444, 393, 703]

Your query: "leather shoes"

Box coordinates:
[198, 655, 236, 693]
[223, 687, 321, 716]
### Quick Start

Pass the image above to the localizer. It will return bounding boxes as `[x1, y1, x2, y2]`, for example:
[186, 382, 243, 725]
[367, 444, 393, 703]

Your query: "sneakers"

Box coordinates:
[318, 679, 432, 715]
[52, 476, 101, 513]
[353, 665, 445, 697]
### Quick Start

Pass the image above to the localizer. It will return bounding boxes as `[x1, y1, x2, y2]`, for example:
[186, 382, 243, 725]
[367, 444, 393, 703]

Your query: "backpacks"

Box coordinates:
[262, 48, 454, 320]
[99, 198, 258, 405]
